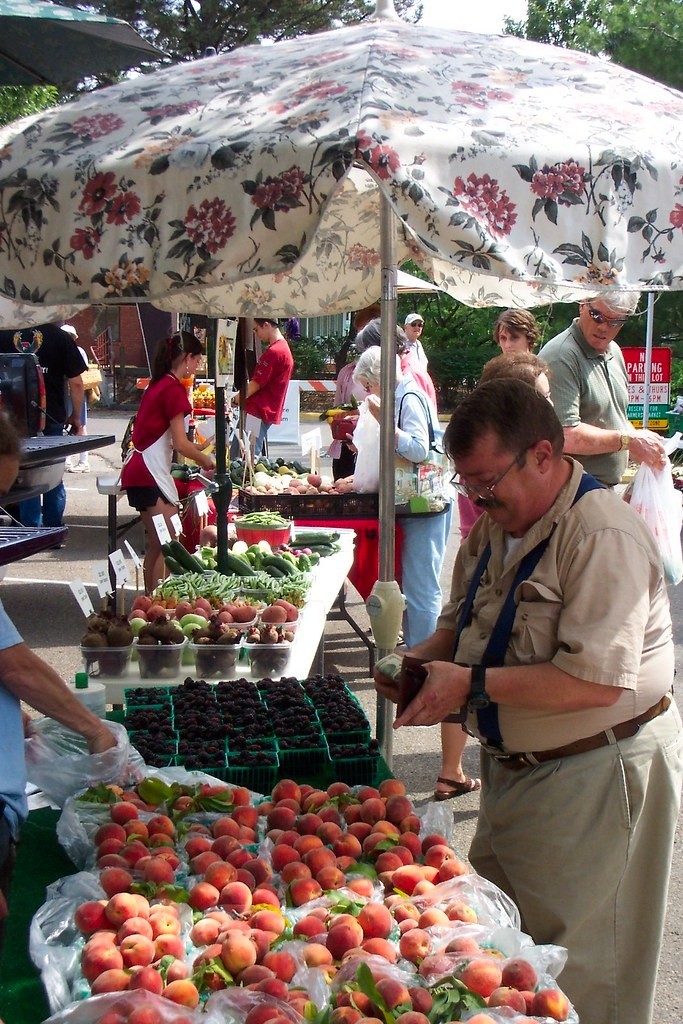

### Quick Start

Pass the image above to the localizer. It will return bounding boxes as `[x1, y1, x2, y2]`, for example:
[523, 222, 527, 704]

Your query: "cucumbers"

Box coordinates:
[160, 539, 321, 578]
[229, 456, 312, 484]
[291, 531, 341, 556]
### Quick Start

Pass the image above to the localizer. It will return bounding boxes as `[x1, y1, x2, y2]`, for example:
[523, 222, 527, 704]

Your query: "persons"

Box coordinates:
[432, 350, 555, 800]
[330, 313, 453, 652]
[0, 319, 88, 548]
[231, 318, 292, 463]
[536, 293, 668, 497]
[116, 330, 216, 603]
[489, 308, 538, 356]
[393, 378, 682, 1024]
[0, 410, 117, 961]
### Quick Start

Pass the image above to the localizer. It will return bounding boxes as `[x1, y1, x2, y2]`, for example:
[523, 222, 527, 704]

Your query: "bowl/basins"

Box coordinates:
[79, 570, 313, 677]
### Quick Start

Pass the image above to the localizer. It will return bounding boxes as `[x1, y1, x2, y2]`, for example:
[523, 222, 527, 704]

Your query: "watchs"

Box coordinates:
[619, 429, 629, 453]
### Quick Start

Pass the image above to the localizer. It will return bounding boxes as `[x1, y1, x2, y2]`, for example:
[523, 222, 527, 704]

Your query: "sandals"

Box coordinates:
[434, 776, 481, 799]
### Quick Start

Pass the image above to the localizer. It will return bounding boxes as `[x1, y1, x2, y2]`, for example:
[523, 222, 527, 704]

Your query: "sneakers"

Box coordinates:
[68, 459, 90, 473]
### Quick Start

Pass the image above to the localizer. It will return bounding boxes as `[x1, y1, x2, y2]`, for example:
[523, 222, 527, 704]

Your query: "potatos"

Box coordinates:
[78, 611, 295, 677]
[131, 595, 299, 631]
[243, 474, 376, 513]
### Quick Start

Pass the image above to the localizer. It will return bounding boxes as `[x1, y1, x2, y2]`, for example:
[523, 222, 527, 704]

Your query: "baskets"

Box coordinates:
[123, 678, 382, 797]
[235, 518, 290, 546]
[238, 488, 379, 520]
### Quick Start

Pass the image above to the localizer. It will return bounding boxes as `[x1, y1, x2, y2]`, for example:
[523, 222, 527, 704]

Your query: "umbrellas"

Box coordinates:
[1, 0, 172, 87]
[0, 0, 682, 773]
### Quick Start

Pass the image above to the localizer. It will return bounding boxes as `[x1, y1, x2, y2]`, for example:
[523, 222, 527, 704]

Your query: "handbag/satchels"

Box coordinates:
[629, 458, 683, 586]
[88, 384, 100, 404]
[393, 392, 450, 519]
[351, 396, 379, 494]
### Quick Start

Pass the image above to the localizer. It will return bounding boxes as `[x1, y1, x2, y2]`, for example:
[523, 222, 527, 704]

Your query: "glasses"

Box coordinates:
[364, 382, 371, 393]
[408, 322, 423, 327]
[450, 446, 530, 499]
[588, 301, 628, 327]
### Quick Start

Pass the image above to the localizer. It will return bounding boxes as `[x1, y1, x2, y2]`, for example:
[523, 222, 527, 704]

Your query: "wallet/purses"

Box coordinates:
[397, 656, 467, 722]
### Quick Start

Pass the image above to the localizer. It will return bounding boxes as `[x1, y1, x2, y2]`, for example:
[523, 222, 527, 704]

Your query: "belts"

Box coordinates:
[487, 686, 673, 769]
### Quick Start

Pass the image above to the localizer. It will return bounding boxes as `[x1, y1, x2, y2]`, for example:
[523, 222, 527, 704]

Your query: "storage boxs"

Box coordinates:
[78, 526, 358, 705]
[238, 484, 379, 520]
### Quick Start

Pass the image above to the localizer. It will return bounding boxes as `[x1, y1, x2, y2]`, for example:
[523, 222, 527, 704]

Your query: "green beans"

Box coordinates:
[239, 511, 289, 525]
[158, 572, 311, 595]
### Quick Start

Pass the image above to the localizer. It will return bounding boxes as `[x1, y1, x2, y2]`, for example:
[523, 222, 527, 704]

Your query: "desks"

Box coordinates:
[97, 474, 157, 616]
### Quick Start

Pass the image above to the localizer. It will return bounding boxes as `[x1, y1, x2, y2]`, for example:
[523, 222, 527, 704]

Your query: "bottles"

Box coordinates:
[187, 420, 195, 443]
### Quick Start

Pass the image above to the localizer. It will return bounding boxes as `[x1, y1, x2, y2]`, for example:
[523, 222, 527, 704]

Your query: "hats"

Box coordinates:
[405, 313, 424, 325]
[60, 325, 78, 339]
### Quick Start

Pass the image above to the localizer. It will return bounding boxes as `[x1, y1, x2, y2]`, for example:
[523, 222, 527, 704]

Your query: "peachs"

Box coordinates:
[75, 778, 569, 1024]
